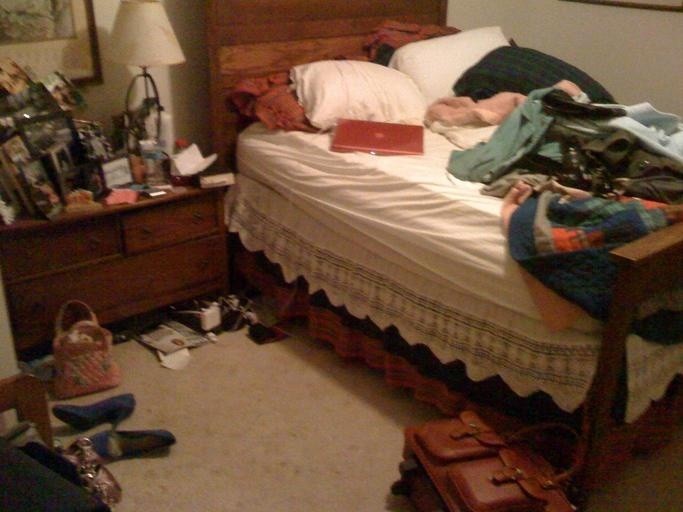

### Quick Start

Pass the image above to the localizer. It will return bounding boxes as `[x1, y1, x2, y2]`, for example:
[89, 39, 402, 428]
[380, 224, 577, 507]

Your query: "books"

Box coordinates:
[195, 157, 235, 189]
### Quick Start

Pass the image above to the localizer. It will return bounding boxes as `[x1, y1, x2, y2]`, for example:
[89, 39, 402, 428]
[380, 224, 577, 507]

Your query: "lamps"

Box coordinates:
[103, 0, 186, 149]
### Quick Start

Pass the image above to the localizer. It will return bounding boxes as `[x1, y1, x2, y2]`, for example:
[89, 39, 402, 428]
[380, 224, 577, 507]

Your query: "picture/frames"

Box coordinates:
[1, 108, 138, 214]
[0, 0, 101, 86]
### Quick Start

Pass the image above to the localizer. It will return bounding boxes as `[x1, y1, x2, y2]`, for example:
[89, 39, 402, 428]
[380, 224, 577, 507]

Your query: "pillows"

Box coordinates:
[389, 26, 512, 106]
[228, 49, 377, 133]
[362, 19, 462, 65]
[286, 55, 425, 132]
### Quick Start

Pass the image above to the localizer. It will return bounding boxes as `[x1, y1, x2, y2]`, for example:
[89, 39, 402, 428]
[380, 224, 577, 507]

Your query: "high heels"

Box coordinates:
[89, 427, 177, 460]
[49, 391, 138, 432]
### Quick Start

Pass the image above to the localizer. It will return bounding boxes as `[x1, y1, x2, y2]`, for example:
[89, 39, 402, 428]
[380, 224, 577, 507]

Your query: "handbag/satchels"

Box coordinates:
[397, 406, 586, 511]
[51, 300, 122, 399]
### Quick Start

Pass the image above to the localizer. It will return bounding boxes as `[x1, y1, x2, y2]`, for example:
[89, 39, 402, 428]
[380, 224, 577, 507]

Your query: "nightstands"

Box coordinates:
[2, 176, 233, 352]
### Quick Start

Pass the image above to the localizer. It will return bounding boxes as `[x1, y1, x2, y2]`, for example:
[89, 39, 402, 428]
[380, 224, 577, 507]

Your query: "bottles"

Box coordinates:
[171, 138, 188, 184]
[139, 140, 161, 186]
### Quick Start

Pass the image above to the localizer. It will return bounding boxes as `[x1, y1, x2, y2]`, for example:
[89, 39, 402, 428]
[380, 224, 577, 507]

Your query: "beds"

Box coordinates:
[202, 0, 682, 489]
